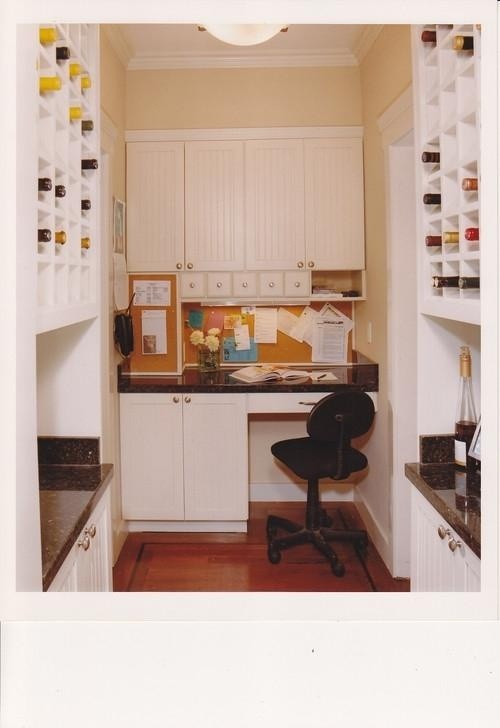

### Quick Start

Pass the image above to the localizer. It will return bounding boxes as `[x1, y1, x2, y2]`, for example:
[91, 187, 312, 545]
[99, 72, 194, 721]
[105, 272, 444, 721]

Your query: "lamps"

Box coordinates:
[197, 22, 289, 46]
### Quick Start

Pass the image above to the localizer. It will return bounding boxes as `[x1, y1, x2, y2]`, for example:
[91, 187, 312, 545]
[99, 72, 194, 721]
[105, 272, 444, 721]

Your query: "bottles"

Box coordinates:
[453, 469, 477, 529]
[454, 346, 477, 472]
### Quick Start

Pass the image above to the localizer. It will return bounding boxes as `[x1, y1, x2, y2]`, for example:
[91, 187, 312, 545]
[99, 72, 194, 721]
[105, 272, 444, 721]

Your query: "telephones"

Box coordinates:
[116, 314, 134, 356]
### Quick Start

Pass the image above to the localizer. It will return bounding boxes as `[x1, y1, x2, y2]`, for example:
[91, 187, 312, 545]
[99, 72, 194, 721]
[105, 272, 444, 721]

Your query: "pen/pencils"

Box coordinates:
[318, 374, 326, 379]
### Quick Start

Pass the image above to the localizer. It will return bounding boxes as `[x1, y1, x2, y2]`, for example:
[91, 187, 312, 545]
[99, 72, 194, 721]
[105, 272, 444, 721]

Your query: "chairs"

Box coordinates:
[266, 390, 374, 577]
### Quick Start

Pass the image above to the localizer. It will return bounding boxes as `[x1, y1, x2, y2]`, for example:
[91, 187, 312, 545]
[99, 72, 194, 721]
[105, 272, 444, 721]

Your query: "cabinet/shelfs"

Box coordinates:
[16, 23, 114, 591]
[409, 25, 481, 590]
[118, 391, 378, 534]
[123, 126, 366, 376]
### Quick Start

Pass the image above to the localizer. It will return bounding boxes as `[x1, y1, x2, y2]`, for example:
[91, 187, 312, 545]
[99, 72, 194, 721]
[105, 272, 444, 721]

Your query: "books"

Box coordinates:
[229, 365, 311, 383]
